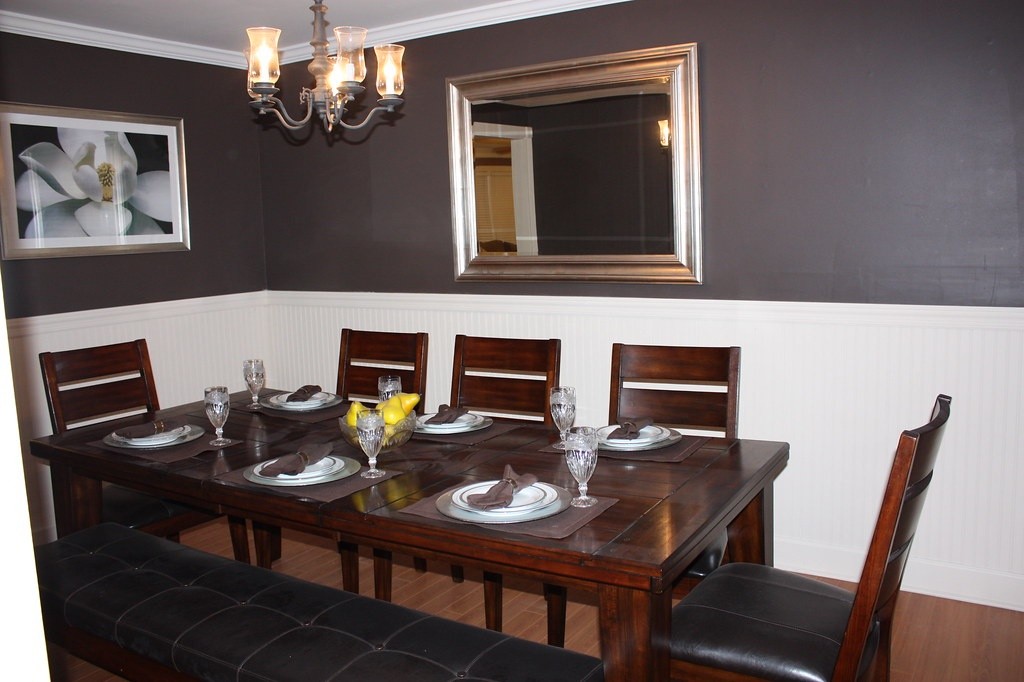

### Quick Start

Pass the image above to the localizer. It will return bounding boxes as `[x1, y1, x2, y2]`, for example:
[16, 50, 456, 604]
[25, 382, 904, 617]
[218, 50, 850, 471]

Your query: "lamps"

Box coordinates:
[242, 1, 405, 144]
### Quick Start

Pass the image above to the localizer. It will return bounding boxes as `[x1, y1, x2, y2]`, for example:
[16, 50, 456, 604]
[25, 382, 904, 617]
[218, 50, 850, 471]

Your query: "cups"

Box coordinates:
[378, 375, 402, 402]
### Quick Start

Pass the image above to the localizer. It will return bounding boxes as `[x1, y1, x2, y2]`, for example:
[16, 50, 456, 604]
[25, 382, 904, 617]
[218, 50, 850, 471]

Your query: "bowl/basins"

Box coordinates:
[338, 410, 416, 453]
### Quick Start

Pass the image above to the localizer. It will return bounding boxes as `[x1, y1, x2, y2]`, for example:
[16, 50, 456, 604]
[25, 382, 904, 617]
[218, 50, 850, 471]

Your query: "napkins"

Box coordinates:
[424, 402, 467, 426]
[286, 384, 323, 403]
[605, 416, 653, 440]
[114, 411, 190, 437]
[260, 439, 336, 477]
[466, 463, 539, 513]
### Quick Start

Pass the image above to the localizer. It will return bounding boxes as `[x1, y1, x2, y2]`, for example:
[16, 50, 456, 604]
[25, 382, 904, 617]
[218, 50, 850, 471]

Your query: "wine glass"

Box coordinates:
[204, 386, 231, 447]
[549, 386, 576, 449]
[243, 359, 265, 409]
[564, 426, 598, 507]
[356, 408, 386, 478]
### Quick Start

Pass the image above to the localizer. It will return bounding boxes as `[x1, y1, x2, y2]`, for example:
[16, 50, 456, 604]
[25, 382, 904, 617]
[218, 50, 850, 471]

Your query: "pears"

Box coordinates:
[345, 393, 422, 444]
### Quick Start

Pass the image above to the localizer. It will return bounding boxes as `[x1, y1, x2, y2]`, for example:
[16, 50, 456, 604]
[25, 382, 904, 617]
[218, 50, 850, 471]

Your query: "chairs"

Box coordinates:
[246, 327, 429, 599]
[659, 393, 957, 682]
[366, 329, 560, 631]
[38, 339, 249, 563]
[591, 344, 743, 596]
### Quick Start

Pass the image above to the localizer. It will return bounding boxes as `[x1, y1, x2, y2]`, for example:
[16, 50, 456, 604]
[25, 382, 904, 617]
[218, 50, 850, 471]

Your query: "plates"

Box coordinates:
[253, 456, 346, 480]
[416, 413, 484, 431]
[449, 480, 559, 517]
[110, 425, 191, 445]
[269, 392, 335, 408]
[596, 423, 670, 447]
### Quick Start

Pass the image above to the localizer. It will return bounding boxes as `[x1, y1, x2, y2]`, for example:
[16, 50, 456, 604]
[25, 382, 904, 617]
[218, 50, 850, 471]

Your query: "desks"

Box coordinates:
[31, 386, 791, 682]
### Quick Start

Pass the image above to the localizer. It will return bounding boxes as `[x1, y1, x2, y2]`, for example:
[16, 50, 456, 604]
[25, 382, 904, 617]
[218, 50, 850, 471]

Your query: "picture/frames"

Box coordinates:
[0, 101, 191, 260]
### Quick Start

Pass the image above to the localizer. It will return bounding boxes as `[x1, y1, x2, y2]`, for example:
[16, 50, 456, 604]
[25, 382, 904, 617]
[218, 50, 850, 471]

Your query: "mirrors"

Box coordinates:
[444, 43, 702, 284]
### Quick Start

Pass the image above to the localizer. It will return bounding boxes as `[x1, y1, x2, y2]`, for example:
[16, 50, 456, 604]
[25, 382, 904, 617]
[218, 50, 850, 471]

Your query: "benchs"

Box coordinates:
[35, 520, 606, 682]
